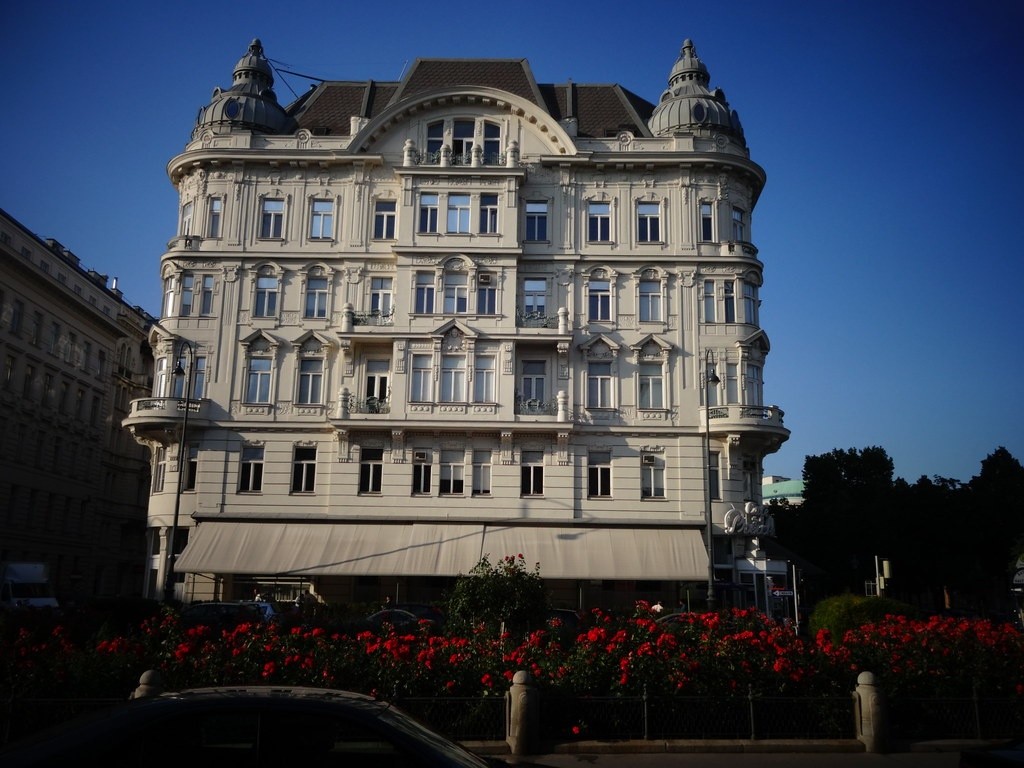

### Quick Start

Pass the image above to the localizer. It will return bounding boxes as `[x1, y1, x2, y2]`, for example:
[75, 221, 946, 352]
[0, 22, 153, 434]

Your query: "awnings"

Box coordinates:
[172, 518, 716, 583]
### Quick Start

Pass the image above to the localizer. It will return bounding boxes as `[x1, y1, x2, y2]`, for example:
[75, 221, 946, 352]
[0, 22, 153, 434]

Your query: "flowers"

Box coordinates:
[1, 550, 1024, 738]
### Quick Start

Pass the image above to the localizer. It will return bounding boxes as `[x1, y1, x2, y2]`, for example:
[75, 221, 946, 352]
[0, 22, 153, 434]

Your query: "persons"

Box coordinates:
[304, 589, 315, 607]
[652, 600, 664, 613]
[254, 591, 267, 602]
[380, 594, 392, 611]
[677, 599, 687, 612]
[284, 708, 338, 767]
[294, 594, 305, 607]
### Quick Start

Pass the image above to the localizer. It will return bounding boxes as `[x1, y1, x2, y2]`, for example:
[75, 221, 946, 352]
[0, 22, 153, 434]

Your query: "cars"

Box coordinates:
[365, 605, 441, 628]
[107, 684, 511, 768]
[244, 601, 300, 624]
[183, 600, 266, 632]
[655, 613, 703, 626]
[514, 607, 586, 638]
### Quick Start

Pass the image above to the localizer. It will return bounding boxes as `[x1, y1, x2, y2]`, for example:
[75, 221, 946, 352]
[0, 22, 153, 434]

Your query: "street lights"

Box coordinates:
[706, 348, 720, 613]
[156, 341, 193, 605]
